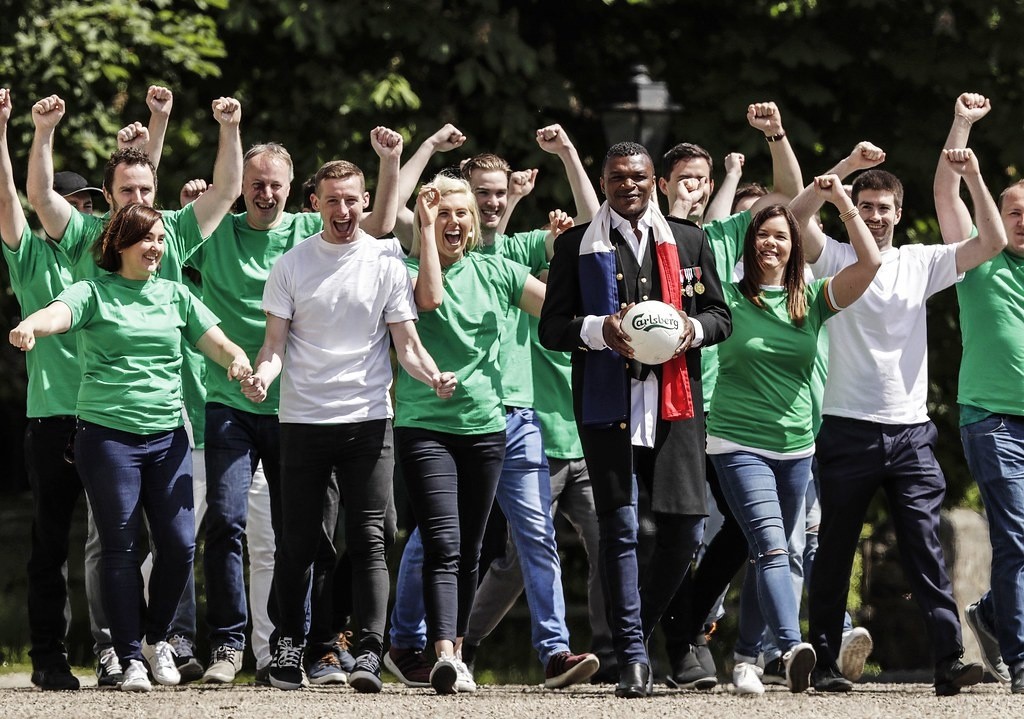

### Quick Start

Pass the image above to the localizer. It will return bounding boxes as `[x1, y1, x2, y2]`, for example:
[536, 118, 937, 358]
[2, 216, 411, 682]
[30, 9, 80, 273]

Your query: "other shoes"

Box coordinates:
[30, 626, 1023, 697]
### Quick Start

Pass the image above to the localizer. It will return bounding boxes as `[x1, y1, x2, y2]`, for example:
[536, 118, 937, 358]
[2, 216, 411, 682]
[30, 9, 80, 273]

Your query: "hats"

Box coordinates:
[52, 171, 105, 198]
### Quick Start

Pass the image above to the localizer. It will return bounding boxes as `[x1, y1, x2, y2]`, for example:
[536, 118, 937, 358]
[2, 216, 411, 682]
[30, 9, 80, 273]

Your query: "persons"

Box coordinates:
[23, 91, 245, 690]
[177, 126, 404, 687]
[297, 171, 406, 671]
[491, 167, 624, 688]
[239, 159, 460, 692]
[705, 98, 879, 702]
[178, 173, 303, 687]
[7, 199, 255, 694]
[780, 140, 1010, 699]
[932, 92, 1022, 698]
[656, 141, 754, 693]
[378, 121, 601, 692]
[377, 168, 578, 695]
[538, 143, 735, 700]
[0, 84, 175, 693]
[702, 173, 887, 700]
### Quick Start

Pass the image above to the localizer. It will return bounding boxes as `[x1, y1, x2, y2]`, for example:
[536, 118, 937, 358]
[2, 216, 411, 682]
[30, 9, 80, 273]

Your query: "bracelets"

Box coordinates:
[766, 129, 786, 142]
[837, 205, 861, 223]
[953, 112, 974, 127]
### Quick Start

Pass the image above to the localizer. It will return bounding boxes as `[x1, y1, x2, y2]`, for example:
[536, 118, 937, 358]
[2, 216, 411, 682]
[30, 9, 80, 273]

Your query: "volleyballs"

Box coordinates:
[621, 299, 687, 367]
